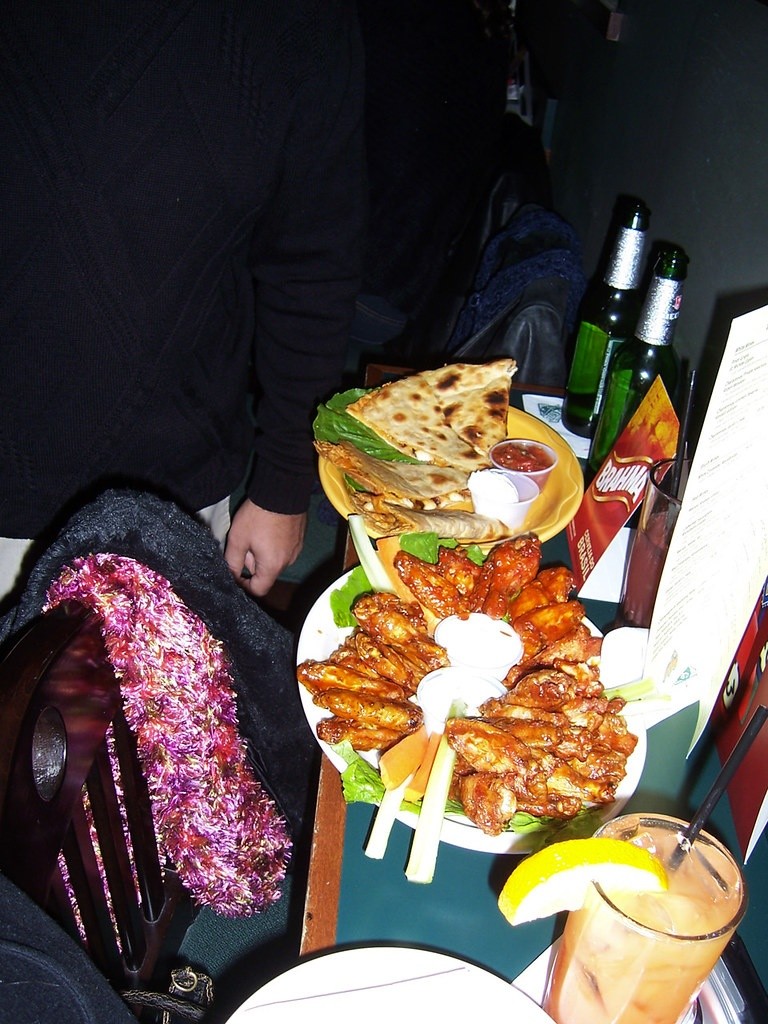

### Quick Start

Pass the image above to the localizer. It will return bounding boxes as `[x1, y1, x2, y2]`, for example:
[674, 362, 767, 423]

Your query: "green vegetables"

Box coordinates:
[314, 386, 422, 492]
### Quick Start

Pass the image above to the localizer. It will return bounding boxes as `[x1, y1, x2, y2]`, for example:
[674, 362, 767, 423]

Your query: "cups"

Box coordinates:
[540, 812, 744, 1024]
[600, 627, 649, 695]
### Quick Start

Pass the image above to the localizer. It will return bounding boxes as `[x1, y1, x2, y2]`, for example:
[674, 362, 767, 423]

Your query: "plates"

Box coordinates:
[297, 406, 650, 855]
[224, 947, 556, 1024]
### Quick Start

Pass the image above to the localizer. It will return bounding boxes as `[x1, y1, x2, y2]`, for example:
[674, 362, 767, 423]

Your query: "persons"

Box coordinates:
[0, 0, 367, 610]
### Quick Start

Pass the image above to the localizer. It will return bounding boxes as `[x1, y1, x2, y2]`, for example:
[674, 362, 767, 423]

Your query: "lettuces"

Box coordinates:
[329, 532, 602, 851]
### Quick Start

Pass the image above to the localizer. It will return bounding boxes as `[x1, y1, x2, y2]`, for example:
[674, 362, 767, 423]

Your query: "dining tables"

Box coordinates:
[298, 365, 768, 1024]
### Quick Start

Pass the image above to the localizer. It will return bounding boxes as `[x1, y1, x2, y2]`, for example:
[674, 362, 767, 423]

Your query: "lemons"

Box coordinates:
[498, 838, 670, 927]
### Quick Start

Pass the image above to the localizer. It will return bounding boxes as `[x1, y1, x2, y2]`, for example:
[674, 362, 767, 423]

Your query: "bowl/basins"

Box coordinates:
[470, 438, 560, 531]
[416, 613, 525, 738]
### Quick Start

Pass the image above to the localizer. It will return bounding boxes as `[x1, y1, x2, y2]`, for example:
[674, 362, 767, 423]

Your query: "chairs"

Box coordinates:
[0, 483, 318, 1024]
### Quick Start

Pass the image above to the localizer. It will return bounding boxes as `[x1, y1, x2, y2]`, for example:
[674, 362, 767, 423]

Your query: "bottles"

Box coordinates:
[559, 196, 688, 489]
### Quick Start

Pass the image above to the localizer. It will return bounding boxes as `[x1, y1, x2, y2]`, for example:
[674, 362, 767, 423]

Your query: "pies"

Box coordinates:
[311, 358, 518, 538]
